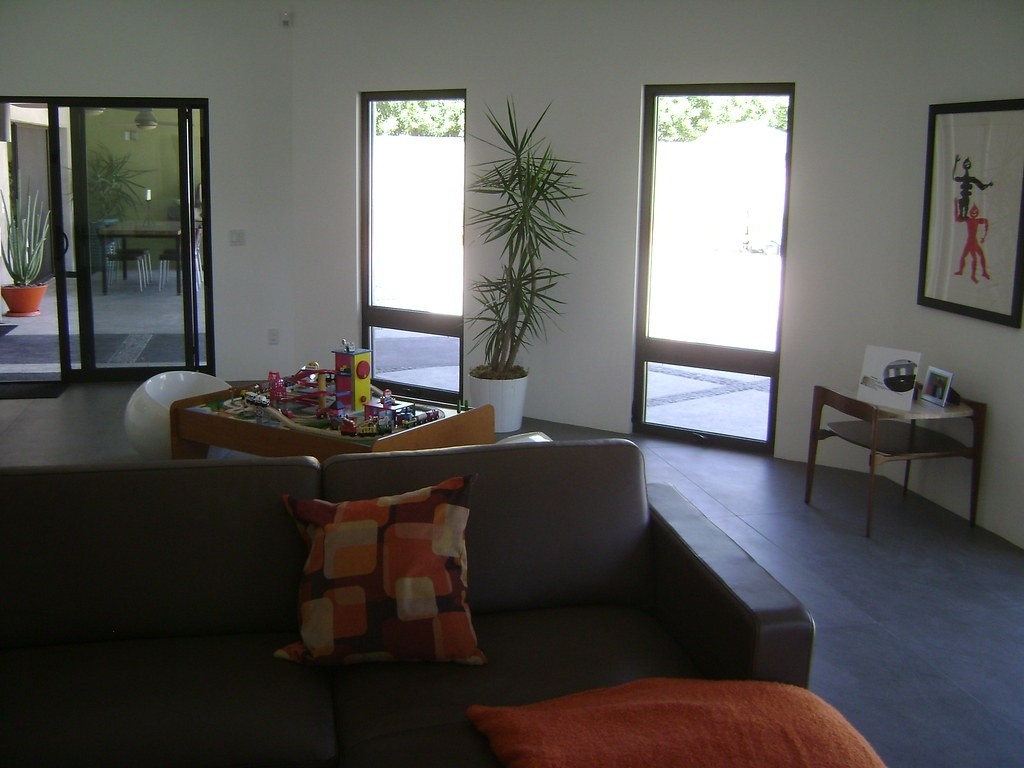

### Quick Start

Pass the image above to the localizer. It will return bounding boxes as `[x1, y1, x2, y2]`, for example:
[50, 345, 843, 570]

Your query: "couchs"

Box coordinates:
[0, 438, 818, 768]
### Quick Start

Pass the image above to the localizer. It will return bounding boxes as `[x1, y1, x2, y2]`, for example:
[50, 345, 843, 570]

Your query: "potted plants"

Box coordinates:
[0, 176, 51, 317]
[62, 142, 154, 272]
[460, 99, 591, 433]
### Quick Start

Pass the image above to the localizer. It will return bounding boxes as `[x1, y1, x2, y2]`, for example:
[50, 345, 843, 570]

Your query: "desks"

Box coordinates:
[169, 379, 496, 464]
[803, 383, 987, 536]
[97, 220, 182, 295]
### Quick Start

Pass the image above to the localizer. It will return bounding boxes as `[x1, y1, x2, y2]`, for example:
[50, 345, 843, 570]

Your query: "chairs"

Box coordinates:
[157, 226, 203, 294]
[124, 370, 236, 460]
[91, 219, 153, 292]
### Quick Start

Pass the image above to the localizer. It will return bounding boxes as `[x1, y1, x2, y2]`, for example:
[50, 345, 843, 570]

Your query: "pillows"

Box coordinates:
[272, 472, 489, 669]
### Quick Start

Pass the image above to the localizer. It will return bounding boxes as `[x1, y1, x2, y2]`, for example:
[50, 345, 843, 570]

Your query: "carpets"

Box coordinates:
[0, 335, 209, 365]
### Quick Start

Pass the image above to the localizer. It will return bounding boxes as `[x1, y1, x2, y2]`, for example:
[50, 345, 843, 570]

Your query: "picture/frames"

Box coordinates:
[917, 99, 1024, 330]
[921, 366, 954, 408]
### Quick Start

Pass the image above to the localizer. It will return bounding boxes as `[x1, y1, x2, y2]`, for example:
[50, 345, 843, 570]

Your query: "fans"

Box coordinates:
[109, 111, 194, 126]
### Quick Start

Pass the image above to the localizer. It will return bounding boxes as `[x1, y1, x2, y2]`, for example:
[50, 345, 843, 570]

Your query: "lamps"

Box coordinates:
[137, 125, 156, 130]
